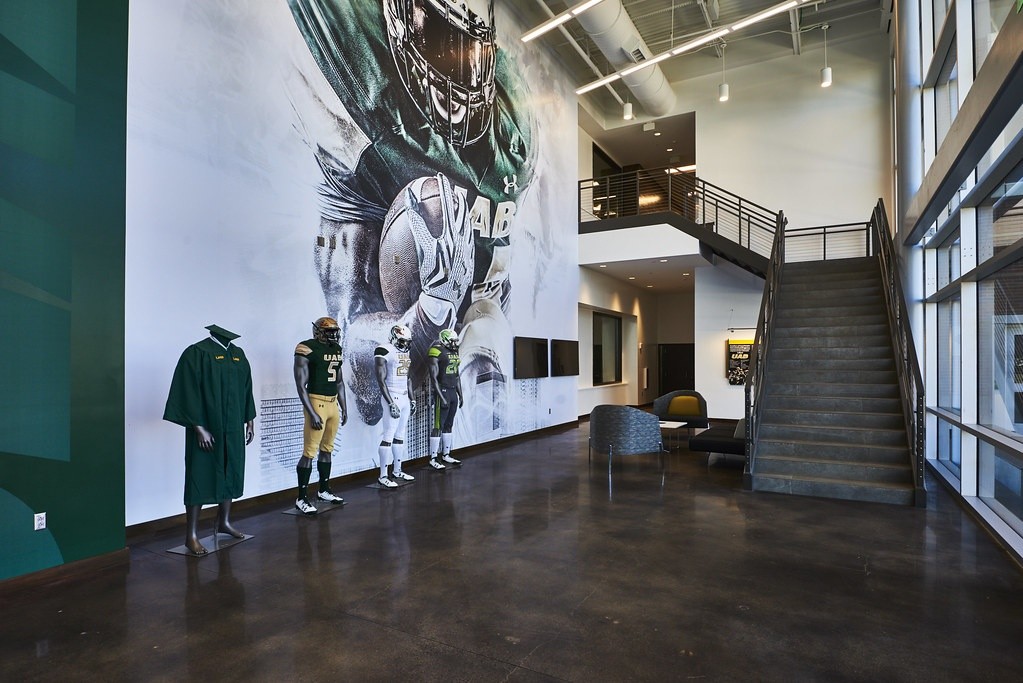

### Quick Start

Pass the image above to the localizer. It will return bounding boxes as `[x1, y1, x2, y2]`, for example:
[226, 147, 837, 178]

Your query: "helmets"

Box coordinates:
[439, 329, 459, 354]
[313, 317, 341, 346]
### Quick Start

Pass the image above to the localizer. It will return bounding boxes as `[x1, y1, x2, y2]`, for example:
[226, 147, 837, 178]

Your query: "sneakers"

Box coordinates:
[430, 459, 445, 469]
[295, 498, 317, 515]
[317, 490, 343, 502]
[378, 477, 398, 487]
[392, 471, 414, 480]
[442, 455, 461, 463]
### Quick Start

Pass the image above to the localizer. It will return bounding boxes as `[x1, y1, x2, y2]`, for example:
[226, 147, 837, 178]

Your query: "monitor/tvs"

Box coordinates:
[515, 337, 548, 378]
[551, 339, 579, 377]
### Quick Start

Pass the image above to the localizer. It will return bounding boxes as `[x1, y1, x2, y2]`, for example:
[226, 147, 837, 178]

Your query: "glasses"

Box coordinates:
[388, 325, 412, 353]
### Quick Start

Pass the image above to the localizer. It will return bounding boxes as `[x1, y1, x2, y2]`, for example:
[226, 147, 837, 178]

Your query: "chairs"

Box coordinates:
[589, 404, 670, 499]
[654, 390, 708, 449]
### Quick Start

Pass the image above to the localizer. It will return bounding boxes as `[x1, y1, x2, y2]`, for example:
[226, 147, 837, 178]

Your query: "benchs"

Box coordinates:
[688, 418, 749, 464]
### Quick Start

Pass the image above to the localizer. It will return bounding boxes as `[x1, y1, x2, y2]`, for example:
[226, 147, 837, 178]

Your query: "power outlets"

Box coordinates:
[34, 512, 47, 530]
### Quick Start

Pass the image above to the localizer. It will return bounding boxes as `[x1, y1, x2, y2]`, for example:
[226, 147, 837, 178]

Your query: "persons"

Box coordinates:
[294, 318, 349, 514]
[284, 0, 535, 424]
[428, 330, 464, 471]
[374, 325, 414, 490]
[163, 321, 257, 555]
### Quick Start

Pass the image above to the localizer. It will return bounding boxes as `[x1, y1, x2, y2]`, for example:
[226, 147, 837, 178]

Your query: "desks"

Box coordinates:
[657, 421, 688, 450]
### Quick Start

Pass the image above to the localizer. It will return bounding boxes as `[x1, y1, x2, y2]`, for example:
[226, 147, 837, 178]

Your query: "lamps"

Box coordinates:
[719, 43, 729, 101]
[623, 85, 632, 120]
[820, 24, 832, 88]
